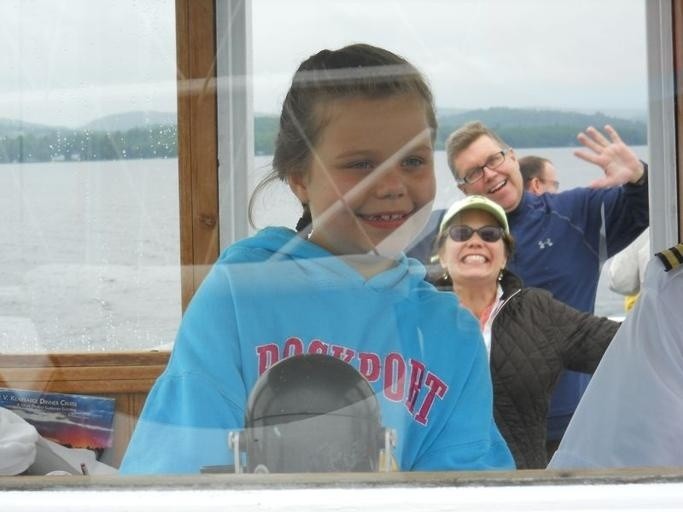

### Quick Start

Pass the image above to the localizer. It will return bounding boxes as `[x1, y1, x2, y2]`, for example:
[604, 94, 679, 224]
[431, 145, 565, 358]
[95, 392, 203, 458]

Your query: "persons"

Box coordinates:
[118, 44, 517, 475]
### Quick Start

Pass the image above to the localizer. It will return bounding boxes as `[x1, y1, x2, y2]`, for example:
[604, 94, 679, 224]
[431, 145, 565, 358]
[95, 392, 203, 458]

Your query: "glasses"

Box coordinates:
[458, 148, 508, 185]
[444, 225, 505, 240]
[528, 177, 559, 191]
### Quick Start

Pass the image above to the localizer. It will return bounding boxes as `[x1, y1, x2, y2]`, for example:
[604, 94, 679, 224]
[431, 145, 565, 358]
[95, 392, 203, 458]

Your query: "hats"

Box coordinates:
[439, 195, 509, 235]
